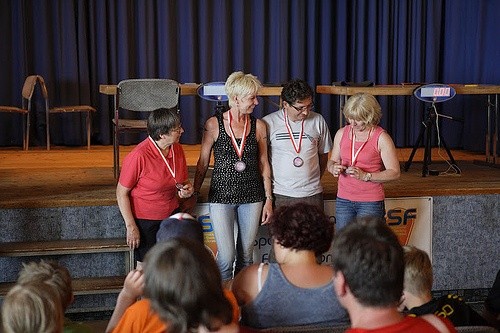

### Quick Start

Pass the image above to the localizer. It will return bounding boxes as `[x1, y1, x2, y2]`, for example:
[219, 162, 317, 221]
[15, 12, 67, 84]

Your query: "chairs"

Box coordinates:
[38, 75, 96, 151]
[112, 78, 180, 186]
[0, 76, 37, 151]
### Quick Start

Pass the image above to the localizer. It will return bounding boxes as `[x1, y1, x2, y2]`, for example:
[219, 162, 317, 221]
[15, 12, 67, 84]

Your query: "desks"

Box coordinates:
[99, 85, 283, 110]
[316, 84, 500, 169]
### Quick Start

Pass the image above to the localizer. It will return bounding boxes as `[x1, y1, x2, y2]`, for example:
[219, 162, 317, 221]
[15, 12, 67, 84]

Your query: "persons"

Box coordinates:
[228, 201, 351, 333]
[262, 79, 334, 264]
[115, 108, 195, 269]
[327, 92, 401, 236]
[179, 71, 274, 296]
[330, 217, 441, 333]
[105, 236, 241, 333]
[155, 212, 205, 246]
[401, 246, 456, 333]
[2, 260, 89, 333]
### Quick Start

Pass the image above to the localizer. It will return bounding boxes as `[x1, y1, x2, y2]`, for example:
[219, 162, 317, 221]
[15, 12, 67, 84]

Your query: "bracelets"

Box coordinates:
[193, 191, 201, 196]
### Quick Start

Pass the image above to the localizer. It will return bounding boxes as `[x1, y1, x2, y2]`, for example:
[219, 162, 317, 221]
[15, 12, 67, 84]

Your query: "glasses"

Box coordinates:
[289, 103, 314, 114]
[171, 124, 183, 134]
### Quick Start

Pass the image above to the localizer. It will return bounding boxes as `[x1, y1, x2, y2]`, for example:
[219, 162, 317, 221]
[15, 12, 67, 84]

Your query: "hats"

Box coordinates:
[156, 213, 205, 247]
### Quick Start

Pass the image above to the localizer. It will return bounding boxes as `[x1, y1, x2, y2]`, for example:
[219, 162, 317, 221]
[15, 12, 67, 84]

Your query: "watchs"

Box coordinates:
[362, 172, 372, 182]
[263, 195, 274, 201]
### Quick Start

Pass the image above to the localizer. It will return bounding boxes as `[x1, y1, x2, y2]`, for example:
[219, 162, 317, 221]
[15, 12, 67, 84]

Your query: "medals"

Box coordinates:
[234, 160, 246, 172]
[174, 182, 185, 192]
[343, 165, 354, 175]
[292, 156, 304, 168]
